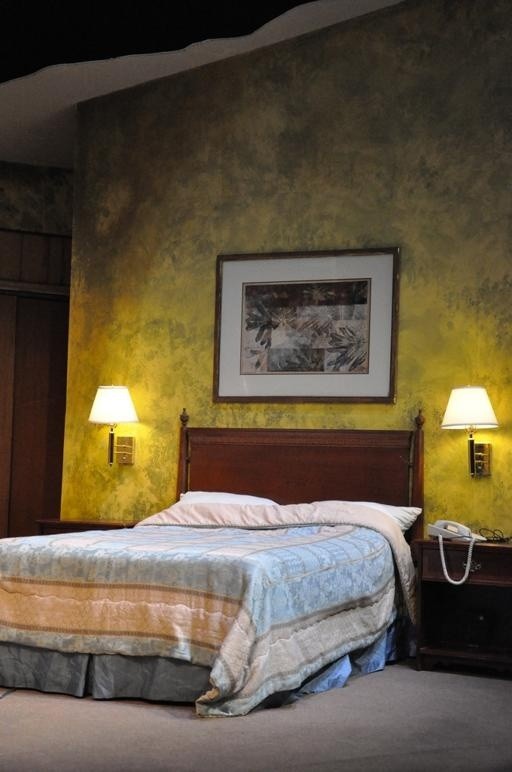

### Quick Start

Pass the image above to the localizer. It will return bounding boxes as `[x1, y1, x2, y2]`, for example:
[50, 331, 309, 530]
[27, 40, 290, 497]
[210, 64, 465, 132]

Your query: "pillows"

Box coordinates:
[179, 491, 278, 509]
[316, 499, 423, 536]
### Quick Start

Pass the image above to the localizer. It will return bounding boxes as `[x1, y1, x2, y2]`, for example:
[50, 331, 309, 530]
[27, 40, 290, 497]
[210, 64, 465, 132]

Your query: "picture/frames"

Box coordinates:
[212, 244, 400, 405]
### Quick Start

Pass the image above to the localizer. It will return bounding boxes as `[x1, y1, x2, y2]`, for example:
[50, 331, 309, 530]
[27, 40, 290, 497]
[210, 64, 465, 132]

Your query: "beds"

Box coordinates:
[0, 405, 433, 716]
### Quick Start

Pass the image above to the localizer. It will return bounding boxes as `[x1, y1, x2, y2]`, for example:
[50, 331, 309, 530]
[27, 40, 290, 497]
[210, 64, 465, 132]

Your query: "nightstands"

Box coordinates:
[412, 535, 512, 682]
[37, 517, 139, 534]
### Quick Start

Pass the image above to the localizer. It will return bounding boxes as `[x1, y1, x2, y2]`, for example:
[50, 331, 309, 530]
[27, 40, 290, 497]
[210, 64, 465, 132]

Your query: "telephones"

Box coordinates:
[427, 520, 487, 543]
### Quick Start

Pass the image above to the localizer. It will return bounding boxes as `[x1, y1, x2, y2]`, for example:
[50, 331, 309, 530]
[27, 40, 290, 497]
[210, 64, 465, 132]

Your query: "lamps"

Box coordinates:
[86, 383, 142, 468]
[439, 385, 502, 481]
